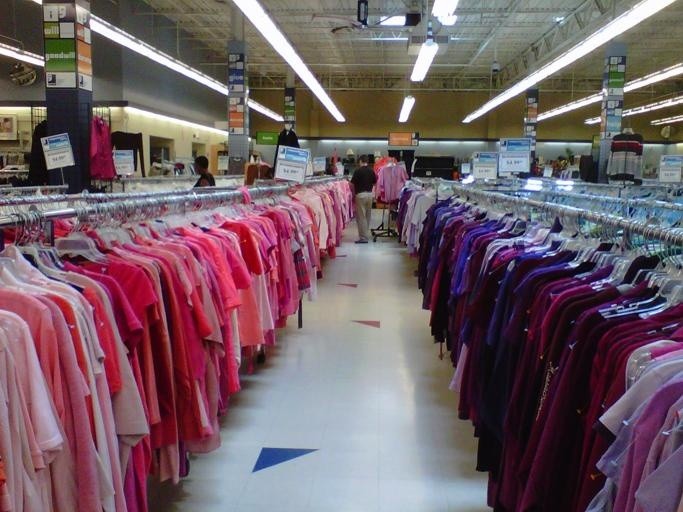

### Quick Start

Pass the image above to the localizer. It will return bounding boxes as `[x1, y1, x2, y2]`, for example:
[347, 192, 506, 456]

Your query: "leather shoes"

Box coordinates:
[355, 240, 368, 243]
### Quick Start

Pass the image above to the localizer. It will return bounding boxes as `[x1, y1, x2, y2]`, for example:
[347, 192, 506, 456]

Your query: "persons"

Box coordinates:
[187, 152, 215, 188]
[273, 121, 300, 172]
[347, 152, 377, 244]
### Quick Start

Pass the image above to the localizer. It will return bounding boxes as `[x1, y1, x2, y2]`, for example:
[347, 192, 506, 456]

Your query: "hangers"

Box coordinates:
[0, 194, 166, 278]
[507, 195, 682, 329]
